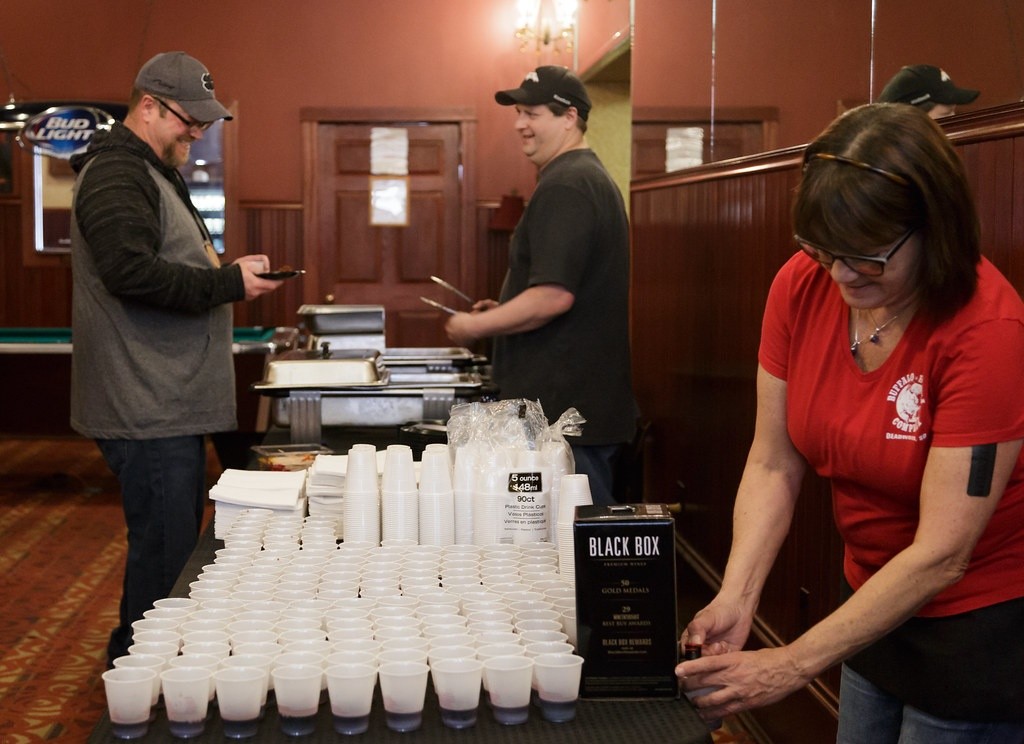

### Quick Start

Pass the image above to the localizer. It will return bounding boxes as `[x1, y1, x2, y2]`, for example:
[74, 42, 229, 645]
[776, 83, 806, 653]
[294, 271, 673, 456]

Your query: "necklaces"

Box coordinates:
[850, 308, 910, 355]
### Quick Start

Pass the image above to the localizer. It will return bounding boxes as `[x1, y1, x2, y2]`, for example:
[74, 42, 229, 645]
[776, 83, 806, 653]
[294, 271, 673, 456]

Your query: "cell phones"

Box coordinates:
[255, 270, 306, 281]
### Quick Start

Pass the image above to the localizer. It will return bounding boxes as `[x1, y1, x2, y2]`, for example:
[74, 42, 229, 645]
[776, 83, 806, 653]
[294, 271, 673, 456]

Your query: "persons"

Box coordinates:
[445, 65, 638, 504]
[70, 51, 285, 669]
[879, 64, 981, 120]
[674, 100, 1024, 744]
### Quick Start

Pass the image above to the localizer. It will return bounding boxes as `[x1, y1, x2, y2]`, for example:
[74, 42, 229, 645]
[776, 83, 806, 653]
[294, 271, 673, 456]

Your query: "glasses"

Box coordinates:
[152, 96, 213, 131]
[794, 231, 914, 276]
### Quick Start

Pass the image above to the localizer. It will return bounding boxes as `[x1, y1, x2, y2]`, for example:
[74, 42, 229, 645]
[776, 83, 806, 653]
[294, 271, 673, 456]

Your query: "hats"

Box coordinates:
[133, 51, 234, 123]
[879, 63, 980, 106]
[494, 66, 592, 122]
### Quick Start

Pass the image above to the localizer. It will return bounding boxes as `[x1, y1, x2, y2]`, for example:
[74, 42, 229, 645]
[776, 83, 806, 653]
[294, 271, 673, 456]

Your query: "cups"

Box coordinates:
[681, 671, 724, 731]
[101, 441, 594, 738]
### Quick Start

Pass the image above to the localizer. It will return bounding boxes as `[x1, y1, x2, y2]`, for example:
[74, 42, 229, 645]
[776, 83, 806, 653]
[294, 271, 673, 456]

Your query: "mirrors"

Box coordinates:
[631, 0, 1024, 177]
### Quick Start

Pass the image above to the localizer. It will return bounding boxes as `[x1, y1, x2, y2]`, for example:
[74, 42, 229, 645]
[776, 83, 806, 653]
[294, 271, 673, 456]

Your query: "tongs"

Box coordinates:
[421, 275, 485, 316]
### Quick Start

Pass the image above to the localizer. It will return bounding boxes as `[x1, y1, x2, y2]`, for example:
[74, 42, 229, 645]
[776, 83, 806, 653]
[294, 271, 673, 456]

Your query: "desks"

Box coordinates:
[85, 423, 712, 744]
[0, 327, 300, 469]
